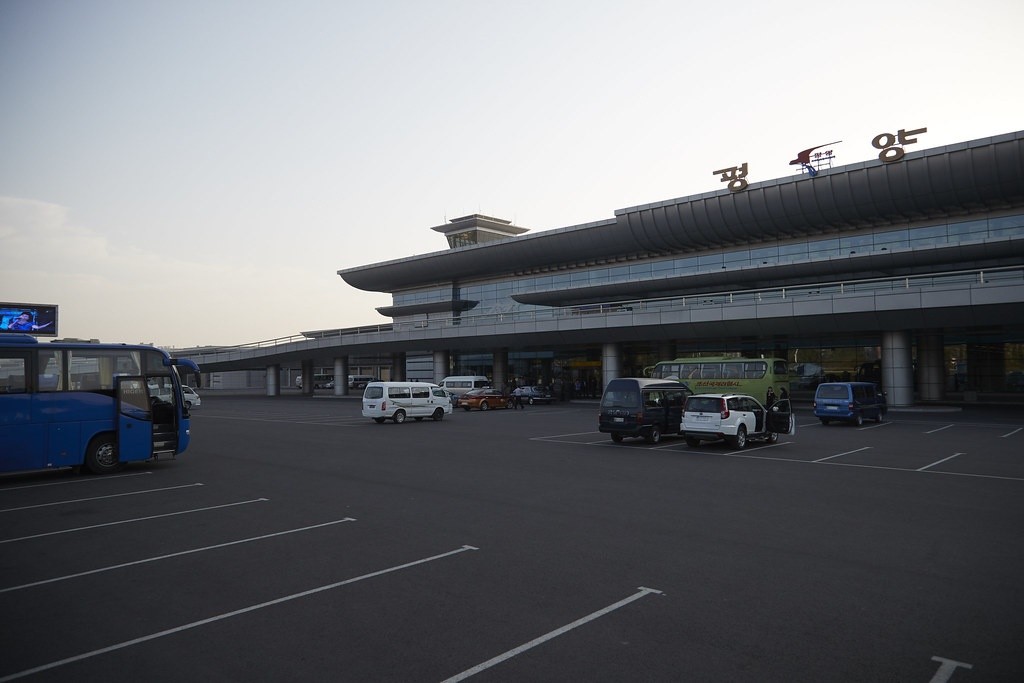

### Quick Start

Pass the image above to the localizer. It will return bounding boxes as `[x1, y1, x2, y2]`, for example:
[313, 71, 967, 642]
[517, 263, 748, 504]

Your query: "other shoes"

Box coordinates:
[522, 407, 524, 409]
[515, 407, 517, 409]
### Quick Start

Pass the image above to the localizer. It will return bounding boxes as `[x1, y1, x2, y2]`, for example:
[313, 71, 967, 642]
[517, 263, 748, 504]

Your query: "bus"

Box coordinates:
[0, 332, 202, 478]
[643, 357, 791, 412]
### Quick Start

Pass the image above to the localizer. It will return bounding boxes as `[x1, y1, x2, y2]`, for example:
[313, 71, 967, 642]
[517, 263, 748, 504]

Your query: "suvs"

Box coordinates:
[679, 393, 795, 450]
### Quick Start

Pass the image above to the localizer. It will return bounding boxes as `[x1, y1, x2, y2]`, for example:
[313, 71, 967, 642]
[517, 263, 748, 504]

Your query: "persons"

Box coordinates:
[8, 311, 53, 332]
[766, 386, 775, 410]
[779, 387, 788, 399]
[513, 385, 524, 410]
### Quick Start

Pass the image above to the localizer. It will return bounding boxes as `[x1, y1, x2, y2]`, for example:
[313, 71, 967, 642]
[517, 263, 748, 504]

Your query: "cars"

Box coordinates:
[511, 386, 551, 405]
[325, 381, 335, 390]
[358, 380, 384, 390]
[458, 385, 515, 411]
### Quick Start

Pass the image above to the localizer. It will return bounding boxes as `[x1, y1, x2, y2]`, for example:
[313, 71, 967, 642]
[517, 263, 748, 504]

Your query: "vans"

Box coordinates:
[148, 384, 201, 410]
[114, 373, 140, 393]
[301, 374, 334, 389]
[438, 375, 489, 399]
[599, 378, 695, 446]
[447, 390, 461, 408]
[813, 382, 888, 427]
[295, 376, 302, 387]
[348, 375, 376, 390]
[361, 381, 453, 424]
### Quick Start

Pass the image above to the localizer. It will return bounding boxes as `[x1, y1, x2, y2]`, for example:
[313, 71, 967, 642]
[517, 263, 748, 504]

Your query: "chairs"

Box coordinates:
[681, 369, 764, 379]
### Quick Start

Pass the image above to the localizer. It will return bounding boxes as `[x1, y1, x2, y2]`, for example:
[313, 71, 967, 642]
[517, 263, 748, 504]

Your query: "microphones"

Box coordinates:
[14, 317, 22, 319]
